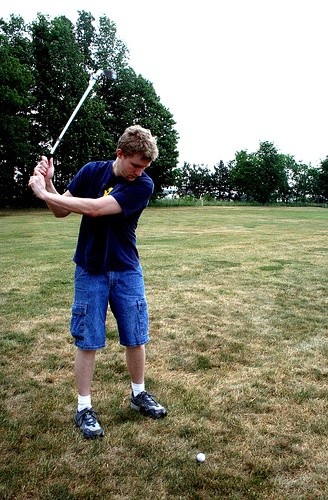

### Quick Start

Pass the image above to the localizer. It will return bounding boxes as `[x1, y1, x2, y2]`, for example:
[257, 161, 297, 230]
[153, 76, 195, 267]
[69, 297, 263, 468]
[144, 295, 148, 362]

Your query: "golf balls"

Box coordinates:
[197, 453, 206, 462]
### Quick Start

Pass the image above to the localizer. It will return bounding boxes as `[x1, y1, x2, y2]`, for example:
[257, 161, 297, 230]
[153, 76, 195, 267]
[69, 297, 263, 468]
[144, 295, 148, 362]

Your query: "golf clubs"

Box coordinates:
[30, 70, 117, 194]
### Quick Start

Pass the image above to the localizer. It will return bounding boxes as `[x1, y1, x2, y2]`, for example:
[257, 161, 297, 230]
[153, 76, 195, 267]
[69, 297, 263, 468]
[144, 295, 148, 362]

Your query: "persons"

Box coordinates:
[28, 125, 168, 438]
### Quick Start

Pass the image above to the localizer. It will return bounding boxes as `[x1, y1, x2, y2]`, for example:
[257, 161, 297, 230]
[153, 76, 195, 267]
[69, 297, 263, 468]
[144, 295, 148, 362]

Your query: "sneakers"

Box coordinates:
[131, 391, 168, 419]
[74, 407, 105, 440]
